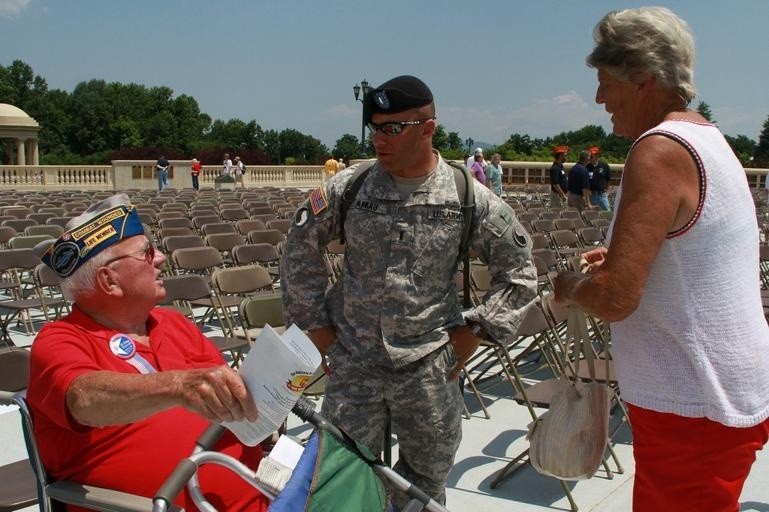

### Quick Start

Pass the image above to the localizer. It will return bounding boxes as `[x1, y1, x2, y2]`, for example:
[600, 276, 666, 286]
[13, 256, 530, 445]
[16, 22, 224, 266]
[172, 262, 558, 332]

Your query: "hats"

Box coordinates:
[587, 146, 600, 156]
[30, 193, 147, 282]
[552, 145, 569, 155]
[362, 75, 435, 127]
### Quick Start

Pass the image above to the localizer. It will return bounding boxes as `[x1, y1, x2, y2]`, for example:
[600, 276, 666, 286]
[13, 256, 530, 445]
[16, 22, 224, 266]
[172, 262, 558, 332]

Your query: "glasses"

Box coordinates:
[100, 240, 155, 274]
[363, 116, 437, 138]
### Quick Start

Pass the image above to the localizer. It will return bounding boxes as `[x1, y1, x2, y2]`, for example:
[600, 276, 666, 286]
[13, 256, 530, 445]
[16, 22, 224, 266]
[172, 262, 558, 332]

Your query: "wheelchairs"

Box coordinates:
[0, 391, 304, 512]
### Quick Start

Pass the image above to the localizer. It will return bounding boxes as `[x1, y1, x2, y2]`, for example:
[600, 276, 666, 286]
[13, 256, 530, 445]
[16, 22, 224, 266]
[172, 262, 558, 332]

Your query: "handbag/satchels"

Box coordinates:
[525, 256, 612, 483]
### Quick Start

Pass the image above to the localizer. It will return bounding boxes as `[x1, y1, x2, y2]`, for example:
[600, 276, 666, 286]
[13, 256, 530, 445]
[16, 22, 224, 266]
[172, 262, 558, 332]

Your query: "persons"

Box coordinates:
[230, 156, 245, 189]
[552, 6, 768, 511]
[279, 74, 537, 511]
[155, 154, 171, 193]
[463, 143, 611, 211]
[323, 154, 339, 179]
[336, 157, 346, 172]
[27, 192, 278, 511]
[191, 157, 201, 190]
[222, 153, 233, 173]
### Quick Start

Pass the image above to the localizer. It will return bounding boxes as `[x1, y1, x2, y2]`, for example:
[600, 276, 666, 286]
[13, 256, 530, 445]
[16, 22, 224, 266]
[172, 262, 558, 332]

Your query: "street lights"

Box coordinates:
[353, 77, 374, 157]
